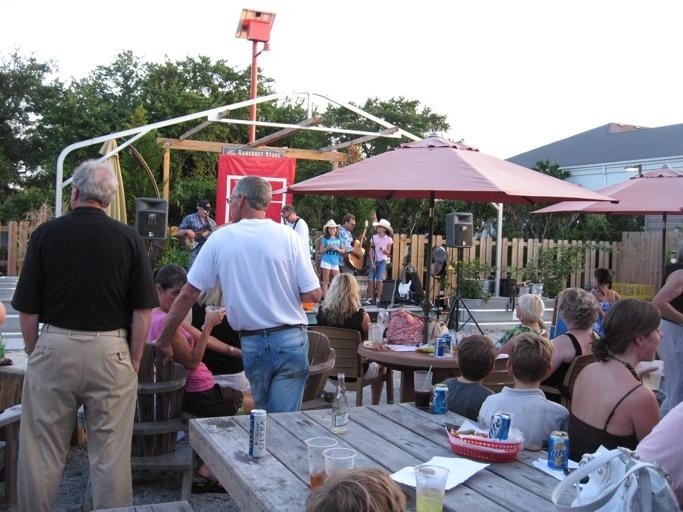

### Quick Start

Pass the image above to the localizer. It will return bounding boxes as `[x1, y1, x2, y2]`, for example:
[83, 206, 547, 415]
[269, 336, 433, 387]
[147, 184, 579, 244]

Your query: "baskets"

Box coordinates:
[449, 432, 521, 462]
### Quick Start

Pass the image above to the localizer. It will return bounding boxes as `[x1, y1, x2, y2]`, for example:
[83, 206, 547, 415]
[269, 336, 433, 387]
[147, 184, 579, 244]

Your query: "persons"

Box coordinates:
[145, 175, 396, 496]
[16, 157, 161, 512]
[303, 466, 407, 512]
[437, 251, 683, 512]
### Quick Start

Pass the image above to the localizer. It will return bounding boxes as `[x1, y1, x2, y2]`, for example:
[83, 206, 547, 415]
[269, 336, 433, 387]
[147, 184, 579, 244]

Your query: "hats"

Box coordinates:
[323, 219, 339, 234]
[372, 219, 393, 234]
[198, 200, 211, 210]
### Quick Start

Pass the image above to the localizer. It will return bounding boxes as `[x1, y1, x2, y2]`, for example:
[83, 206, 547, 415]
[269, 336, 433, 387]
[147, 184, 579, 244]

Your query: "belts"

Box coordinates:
[239, 325, 291, 338]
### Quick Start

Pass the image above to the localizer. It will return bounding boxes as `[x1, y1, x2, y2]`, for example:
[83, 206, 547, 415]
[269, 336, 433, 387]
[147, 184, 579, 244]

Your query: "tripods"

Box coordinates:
[445, 248, 485, 335]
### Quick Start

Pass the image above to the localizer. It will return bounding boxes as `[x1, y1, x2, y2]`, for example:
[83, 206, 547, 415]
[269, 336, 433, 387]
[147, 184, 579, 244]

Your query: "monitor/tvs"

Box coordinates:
[380, 280, 395, 308]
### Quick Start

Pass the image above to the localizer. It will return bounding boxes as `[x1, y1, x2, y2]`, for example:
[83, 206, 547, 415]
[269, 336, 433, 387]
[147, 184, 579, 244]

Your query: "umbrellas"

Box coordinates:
[281, 129, 622, 347]
[97, 132, 128, 226]
[528, 160, 682, 285]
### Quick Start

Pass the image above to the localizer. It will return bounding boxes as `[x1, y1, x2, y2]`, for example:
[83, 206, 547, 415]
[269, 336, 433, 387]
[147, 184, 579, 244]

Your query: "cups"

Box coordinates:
[414, 465, 447, 511]
[639, 359, 664, 390]
[412, 369, 433, 409]
[365, 322, 384, 351]
[205, 306, 221, 326]
[305, 435, 355, 491]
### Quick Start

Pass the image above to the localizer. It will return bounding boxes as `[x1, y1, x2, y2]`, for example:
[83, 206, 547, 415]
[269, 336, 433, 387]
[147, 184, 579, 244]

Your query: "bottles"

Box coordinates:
[332, 372, 349, 438]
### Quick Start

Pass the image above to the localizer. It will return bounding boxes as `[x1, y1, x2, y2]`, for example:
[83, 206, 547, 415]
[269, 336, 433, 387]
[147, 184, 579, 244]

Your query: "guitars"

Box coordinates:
[183, 224, 211, 251]
[344, 219, 370, 270]
[395, 261, 411, 301]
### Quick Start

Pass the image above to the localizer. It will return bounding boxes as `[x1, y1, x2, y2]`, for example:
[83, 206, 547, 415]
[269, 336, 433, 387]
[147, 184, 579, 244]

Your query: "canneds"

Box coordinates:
[442, 334, 452, 353]
[488, 412, 511, 439]
[431, 383, 449, 415]
[248, 409, 265, 461]
[548, 431, 570, 471]
[434, 338, 446, 357]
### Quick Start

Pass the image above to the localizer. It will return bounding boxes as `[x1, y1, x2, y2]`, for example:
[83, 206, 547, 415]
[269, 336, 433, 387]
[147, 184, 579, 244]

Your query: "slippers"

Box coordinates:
[190, 476, 226, 493]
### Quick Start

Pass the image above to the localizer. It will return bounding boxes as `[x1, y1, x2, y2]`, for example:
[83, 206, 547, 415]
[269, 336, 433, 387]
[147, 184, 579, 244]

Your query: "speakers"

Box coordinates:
[500, 278, 516, 296]
[446, 213, 473, 248]
[136, 198, 168, 240]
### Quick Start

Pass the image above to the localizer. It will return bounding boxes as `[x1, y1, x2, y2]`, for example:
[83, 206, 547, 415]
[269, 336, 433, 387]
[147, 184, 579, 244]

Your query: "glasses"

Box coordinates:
[226, 195, 236, 204]
